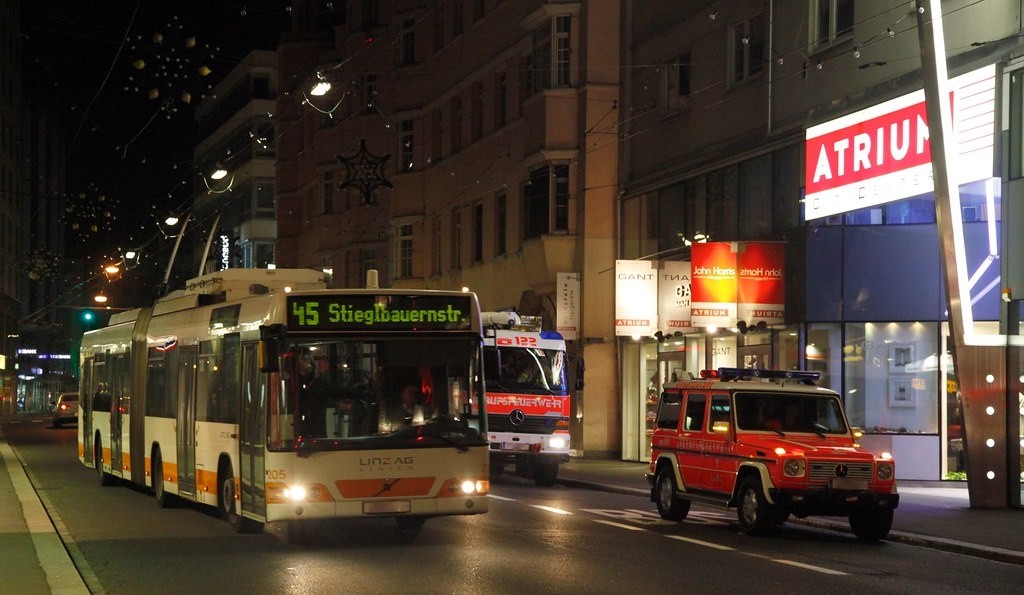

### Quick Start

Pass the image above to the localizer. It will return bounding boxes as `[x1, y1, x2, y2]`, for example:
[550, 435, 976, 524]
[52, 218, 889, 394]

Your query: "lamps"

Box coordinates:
[735, 320, 767, 334]
[654, 330, 683, 342]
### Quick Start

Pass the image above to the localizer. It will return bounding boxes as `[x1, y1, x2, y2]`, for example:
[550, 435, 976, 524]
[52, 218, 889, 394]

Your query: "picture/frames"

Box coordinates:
[888, 341, 918, 374]
[887, 377, 918, 408]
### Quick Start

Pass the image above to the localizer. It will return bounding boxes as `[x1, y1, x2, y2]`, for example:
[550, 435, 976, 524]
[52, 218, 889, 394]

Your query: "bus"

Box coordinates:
[78, 264, 490, 534]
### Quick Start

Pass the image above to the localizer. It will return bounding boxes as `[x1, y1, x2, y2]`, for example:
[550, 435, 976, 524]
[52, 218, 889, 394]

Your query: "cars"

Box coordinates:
[51, 392, 78, 428]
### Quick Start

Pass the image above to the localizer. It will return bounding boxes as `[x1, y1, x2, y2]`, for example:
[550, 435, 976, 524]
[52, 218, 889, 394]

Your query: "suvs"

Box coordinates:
[650, 368, 900, 539]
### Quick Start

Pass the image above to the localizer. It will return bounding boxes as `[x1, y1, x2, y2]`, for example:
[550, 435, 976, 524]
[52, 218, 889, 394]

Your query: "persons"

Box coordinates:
[648, 372, 658, 388]
[782, 403, 800, 432]
[517, 348, 554, 387]
[288, 341, 364, 440]
[93, 383, 111, 411]
[390, 386, 429, 431]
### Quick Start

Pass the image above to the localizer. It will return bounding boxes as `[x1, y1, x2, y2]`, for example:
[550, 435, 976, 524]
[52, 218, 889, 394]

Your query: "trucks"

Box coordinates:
[450, 312, 585, 486]
